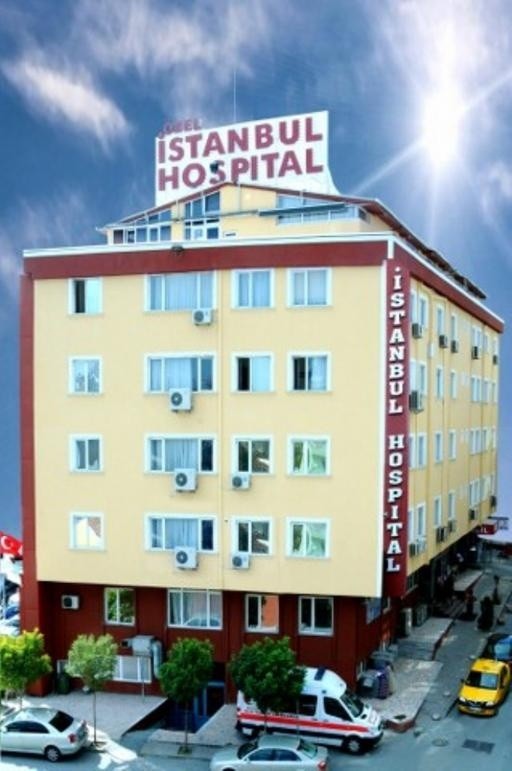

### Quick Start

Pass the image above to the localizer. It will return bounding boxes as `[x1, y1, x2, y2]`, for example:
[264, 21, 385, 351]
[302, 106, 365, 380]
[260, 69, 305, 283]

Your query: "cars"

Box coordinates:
[209, 735, 334, 771]
[0, 707, 88, 762]
[457, 633, 512, 716]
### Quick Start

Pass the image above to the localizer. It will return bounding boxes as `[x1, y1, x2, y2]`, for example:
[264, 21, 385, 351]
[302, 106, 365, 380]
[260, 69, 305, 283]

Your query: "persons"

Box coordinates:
[464, 590, 476, 617]
[375, 668, 389, 700]
[476, 597, 494, 632]
[492, 574, 501, 605]
[384, 660, 396, 696]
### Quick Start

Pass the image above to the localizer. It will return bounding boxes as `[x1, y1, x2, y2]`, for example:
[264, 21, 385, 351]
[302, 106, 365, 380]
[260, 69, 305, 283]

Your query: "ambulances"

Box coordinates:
[234, 665, 384, 756]
